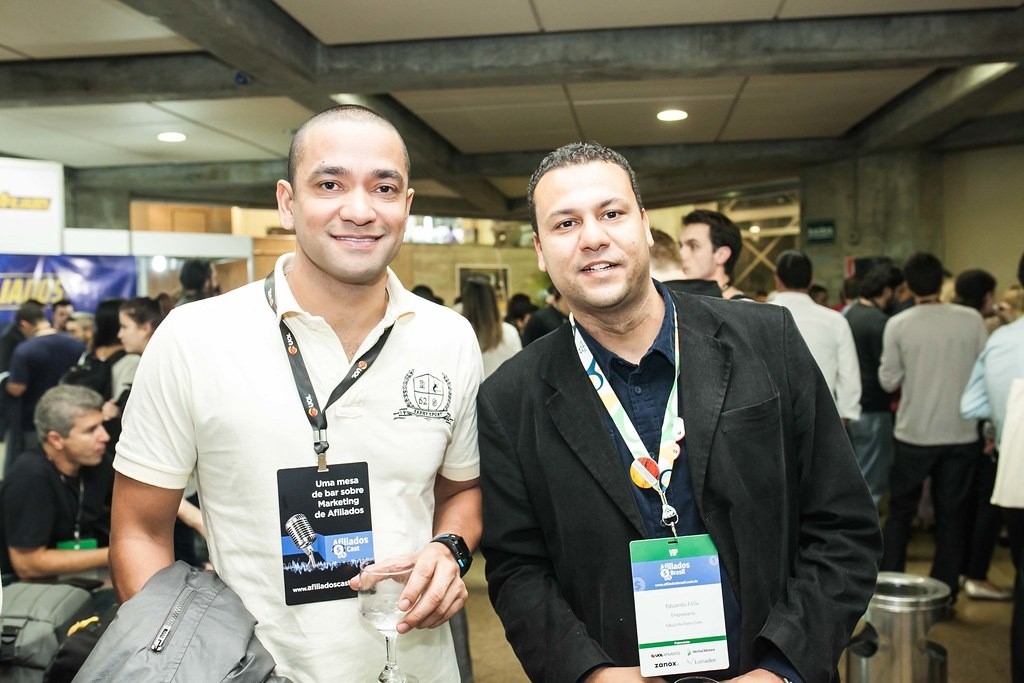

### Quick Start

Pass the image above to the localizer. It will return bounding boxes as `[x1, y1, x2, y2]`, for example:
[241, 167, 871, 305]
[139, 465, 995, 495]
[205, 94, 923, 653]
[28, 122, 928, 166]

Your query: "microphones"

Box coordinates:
[286, 514, 317, 566]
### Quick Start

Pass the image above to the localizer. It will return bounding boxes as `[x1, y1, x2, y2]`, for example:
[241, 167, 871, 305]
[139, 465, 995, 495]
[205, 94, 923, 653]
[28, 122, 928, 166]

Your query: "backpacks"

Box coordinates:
[60, 345, 127, 402]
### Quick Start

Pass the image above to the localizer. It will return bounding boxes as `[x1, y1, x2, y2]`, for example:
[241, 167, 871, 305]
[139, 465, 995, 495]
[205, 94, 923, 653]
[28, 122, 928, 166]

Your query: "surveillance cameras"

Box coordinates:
[232, 70, 252, 86]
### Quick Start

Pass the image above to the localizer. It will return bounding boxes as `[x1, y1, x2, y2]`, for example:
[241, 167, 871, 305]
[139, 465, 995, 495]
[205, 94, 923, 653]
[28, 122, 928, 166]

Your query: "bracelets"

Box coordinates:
[783, 677, 793, 683]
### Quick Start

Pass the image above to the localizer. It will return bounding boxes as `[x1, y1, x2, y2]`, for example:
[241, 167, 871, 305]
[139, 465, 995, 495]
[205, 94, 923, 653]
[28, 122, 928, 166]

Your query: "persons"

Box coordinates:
[0, 259, 213, 571]
[476, 141, 881, 683]
[454, 275, 524, 380]
[952, 266, 1011, 601]
[413, 283, 570, 349]
[676, 210, 758, 301]
[765, 249, 862, 430]
[875, 250, 995, 616]
[108, 106, 484, 683]
[648, 227, 724, 298]
[806, 264, 1024, 534]
[0, 385, 114, 683]
[957, 253, 1024, 683]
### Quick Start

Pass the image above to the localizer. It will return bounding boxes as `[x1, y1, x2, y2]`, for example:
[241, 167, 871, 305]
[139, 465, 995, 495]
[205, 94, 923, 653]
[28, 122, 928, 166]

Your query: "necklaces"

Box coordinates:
[720, 281, 733, 293]
[31, 326, 52, 335]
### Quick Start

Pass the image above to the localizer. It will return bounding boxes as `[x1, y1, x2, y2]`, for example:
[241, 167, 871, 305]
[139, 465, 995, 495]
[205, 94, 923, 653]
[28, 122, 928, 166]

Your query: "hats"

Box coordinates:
[504, 293, 536, 321]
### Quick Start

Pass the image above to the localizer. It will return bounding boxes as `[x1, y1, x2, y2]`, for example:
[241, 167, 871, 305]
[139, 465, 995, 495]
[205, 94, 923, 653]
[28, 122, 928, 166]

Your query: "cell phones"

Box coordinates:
[65, 577, 104, 590]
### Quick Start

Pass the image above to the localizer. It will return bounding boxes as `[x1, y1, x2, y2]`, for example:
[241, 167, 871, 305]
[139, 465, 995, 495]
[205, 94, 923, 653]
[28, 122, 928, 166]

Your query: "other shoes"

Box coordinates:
[965, 580, 1013, 600]
[959, 576, 967, 587]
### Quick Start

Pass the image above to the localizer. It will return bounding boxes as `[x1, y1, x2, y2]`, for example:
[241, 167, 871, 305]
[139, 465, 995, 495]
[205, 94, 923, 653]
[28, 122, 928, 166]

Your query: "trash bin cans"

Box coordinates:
[846, 573, 952, 683]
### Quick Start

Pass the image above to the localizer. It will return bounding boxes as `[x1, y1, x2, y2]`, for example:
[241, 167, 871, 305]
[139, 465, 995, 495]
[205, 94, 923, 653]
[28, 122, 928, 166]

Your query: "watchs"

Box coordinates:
[429, 533, 473, 578]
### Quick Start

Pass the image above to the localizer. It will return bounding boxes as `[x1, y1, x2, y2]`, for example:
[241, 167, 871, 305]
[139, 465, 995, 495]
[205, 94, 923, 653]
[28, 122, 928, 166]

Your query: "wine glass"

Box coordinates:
[356, 557, 420, 683]
